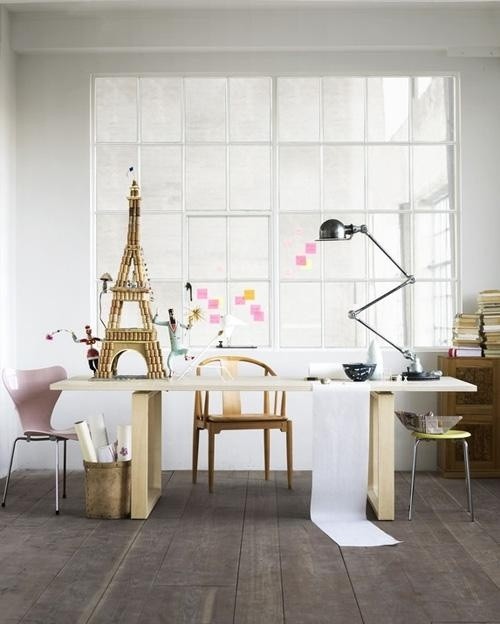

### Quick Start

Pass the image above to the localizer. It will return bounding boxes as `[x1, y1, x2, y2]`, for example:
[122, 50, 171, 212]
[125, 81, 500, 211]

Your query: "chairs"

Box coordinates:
[190, 357, 296, 495]
[1, 365, 87, 514]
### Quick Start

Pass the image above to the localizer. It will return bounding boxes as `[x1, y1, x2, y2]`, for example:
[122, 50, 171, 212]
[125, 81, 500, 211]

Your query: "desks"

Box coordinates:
[51, 374, 477, 521]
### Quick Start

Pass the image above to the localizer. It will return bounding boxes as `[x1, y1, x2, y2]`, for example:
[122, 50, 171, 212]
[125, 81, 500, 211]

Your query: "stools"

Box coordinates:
[409, 429, 474, 522]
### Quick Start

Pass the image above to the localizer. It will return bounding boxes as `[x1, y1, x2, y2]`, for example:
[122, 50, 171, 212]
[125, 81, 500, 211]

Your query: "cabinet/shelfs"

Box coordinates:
[438, 355, 499, 478]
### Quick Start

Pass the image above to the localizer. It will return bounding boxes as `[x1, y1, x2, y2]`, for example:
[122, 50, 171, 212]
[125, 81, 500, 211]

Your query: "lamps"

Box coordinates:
[316, 217, 440, 380]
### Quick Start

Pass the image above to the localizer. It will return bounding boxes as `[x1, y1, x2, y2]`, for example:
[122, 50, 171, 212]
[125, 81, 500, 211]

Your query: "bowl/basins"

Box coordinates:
[342, 362, 376, 383]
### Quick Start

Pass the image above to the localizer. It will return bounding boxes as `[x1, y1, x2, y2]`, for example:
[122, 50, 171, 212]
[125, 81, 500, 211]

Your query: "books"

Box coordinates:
[448, 288, 500, 358]
